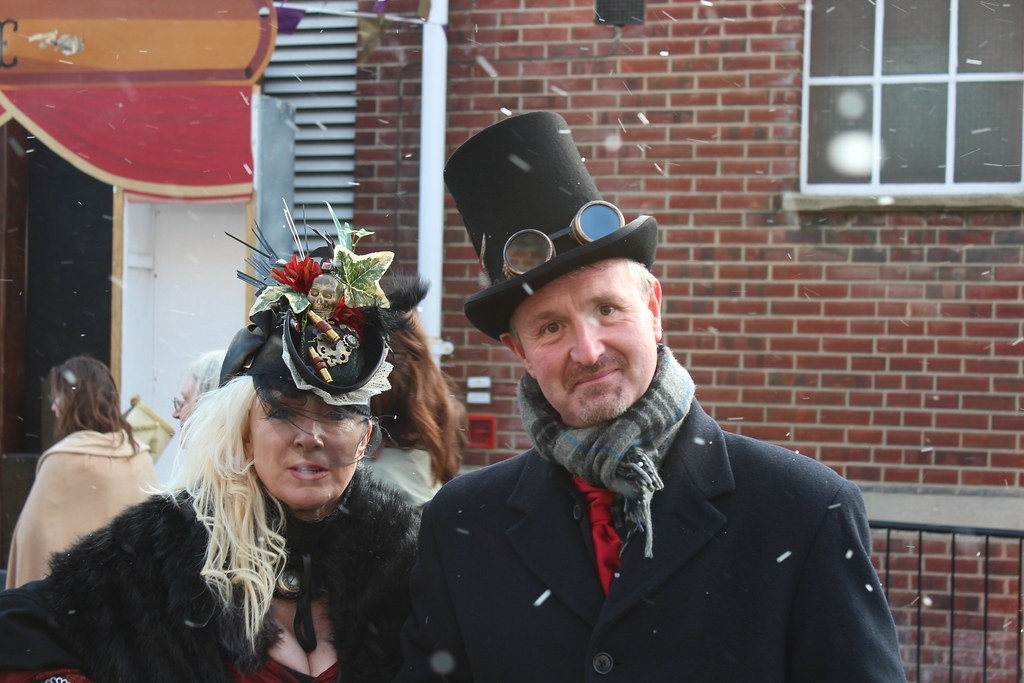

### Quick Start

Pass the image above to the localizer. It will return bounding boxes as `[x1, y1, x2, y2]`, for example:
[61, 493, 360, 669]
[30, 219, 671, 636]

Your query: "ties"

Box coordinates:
[574, 475, 622, 598]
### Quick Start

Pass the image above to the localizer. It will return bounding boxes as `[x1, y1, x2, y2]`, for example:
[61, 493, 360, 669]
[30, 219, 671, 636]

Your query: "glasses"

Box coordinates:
[173, 398, 186, 413]
[491, 200, 625, 287]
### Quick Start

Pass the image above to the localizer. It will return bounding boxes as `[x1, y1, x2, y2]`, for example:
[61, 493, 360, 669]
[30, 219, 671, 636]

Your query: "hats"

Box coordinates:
[442, 110, 659, 343]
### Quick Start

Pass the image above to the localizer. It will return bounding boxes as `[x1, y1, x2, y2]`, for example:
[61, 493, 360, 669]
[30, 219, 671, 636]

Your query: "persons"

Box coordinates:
[396, 241, 907, 683]
[173, 350, 224, 435]
[5, 355, 154, 593]
[358, 296, 466, 538]
[0, 309, 421, 683]
